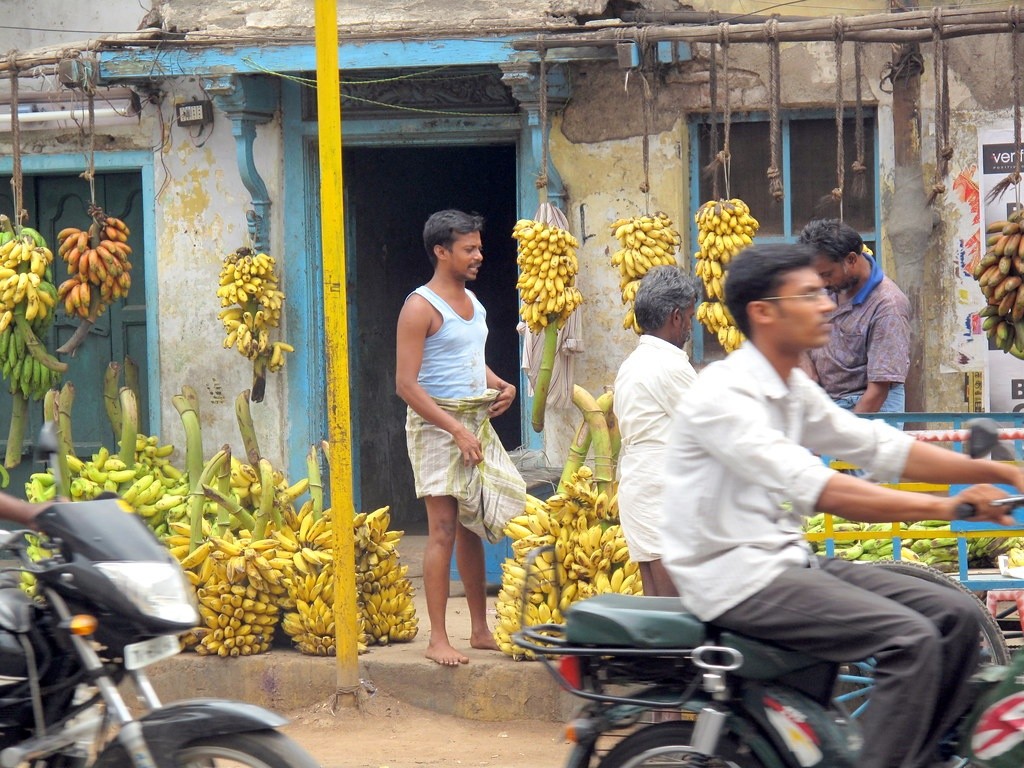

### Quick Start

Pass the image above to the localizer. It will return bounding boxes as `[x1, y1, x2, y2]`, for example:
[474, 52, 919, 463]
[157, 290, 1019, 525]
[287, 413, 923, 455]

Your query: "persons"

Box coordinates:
[0, 492, 56, 525]
[796, 221, 915, 481]
[663, 244, 1024, 768]
[615, 266, 700, 600]
[395, 209, 517, 667]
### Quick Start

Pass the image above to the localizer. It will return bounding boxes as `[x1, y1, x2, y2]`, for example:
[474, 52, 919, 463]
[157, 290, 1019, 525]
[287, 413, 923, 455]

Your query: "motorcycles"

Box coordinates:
[0, 489, 322, 768]
[508, 416, 1024, 768]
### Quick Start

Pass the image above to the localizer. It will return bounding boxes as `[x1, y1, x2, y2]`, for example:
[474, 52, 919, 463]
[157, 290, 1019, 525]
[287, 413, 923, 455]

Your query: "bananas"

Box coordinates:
[0, 211, 420, 656]
[492, 198, 1024, 660]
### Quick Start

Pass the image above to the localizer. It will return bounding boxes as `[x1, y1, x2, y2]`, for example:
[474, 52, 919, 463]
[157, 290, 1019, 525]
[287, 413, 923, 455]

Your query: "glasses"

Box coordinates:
[759, 286, 831, 302]
